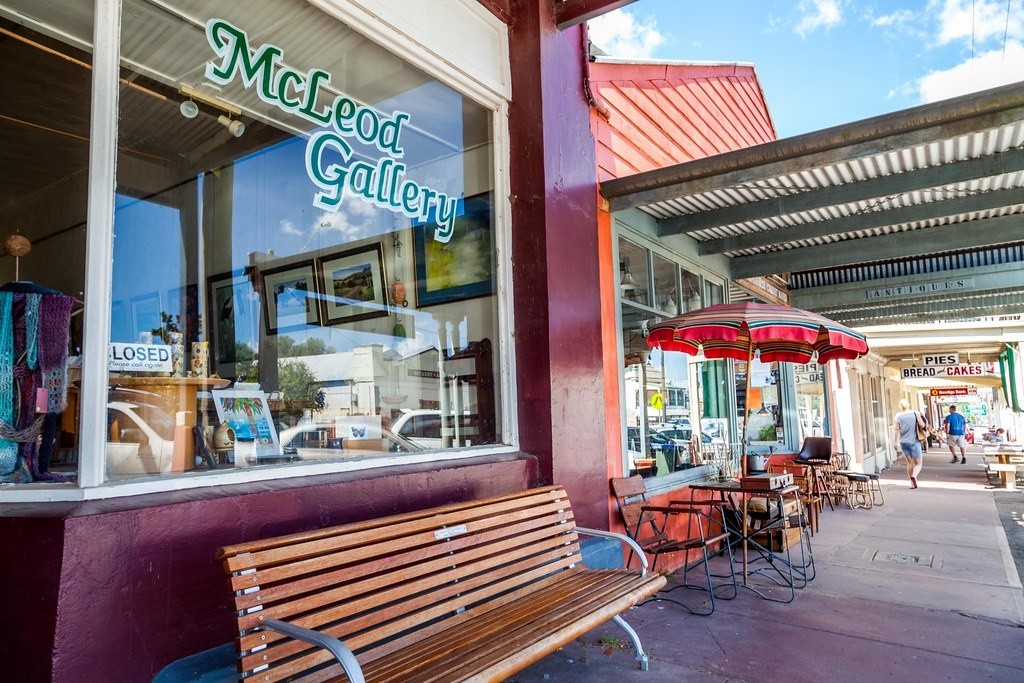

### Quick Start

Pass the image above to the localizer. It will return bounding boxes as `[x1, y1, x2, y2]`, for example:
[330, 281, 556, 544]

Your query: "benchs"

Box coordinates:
[988, 463, 1024, 490]
[215, 485, 668, 683]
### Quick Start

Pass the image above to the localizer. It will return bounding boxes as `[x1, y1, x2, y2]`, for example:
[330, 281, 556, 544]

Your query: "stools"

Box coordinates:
[793, 437, 835, 513]
[834, 469, 883, 510]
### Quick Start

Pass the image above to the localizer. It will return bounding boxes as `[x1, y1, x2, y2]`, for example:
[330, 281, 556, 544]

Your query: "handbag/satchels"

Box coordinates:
[913, 412, 925, 441]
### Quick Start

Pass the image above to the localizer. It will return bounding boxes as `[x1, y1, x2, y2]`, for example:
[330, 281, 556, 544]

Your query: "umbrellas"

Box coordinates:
[646, 299, 869, 587]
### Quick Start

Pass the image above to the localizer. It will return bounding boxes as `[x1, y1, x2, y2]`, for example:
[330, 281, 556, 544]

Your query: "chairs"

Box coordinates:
[611, 474, 738, 616]
[768, 464, 821, 537]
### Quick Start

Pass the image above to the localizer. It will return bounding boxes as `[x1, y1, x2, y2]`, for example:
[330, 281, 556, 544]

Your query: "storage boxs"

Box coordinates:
[728, 499, 802, 553]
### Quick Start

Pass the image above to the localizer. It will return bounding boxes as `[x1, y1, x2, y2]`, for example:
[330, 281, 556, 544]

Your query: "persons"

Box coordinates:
[982, 428, 1004, 443]
[919, 412, 975, 450]
[893, 398, 929, 489]
[945, 405, 967, 464]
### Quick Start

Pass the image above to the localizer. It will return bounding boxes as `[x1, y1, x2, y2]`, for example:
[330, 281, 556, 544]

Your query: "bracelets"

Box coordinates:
[946, 433, 949, 434]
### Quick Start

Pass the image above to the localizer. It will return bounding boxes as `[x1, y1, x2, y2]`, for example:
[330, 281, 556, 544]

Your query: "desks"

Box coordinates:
[986, 452, 1024, 477]
[684, 480, 807, 603]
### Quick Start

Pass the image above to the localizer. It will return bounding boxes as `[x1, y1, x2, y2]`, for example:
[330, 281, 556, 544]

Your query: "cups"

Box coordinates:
[136, 332, 184, 377]
[191, 342, 209, 378]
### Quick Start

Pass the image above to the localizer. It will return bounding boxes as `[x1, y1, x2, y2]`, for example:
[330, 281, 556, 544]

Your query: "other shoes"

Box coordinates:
[950, 455, 958, 463]
[961, 458, 966, 464]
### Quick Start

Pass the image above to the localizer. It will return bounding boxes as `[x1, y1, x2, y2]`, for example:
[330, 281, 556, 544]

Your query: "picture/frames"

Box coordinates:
[258, 258, 321, 336]
[213, 389, 284, 463]
[129, 290, 164, 344]
[411, 189, 497, 309]
[168, 282, 203, 351]
[207, 265, 268, 383]
[111, 300, 127, 342]
[316, 241, 391, 327]
